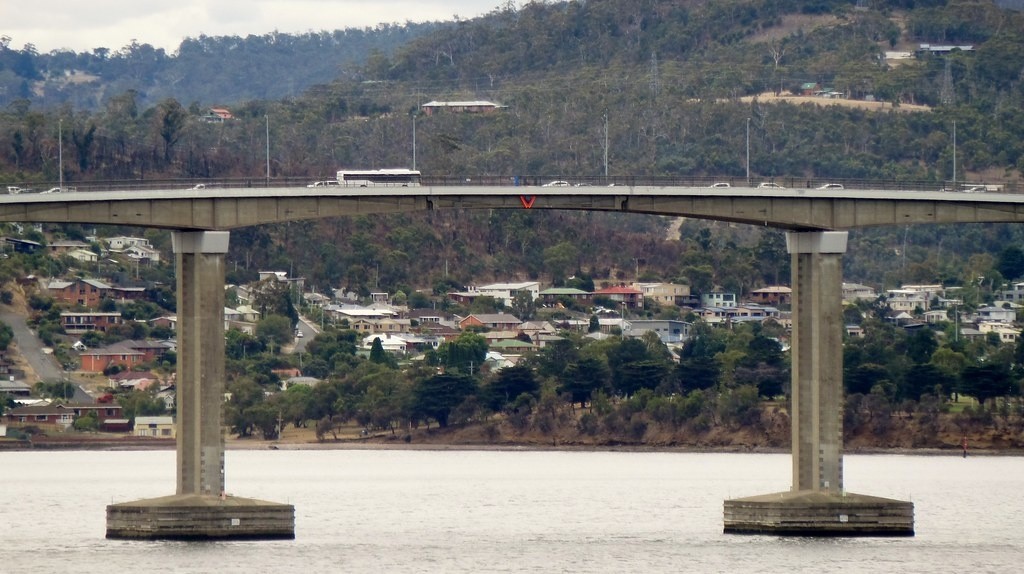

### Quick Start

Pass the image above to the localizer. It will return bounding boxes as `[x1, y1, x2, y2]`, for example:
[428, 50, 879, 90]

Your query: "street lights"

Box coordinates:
[747, 118, 750, 185]
[264, 115, 269, 187]
[952, 120, 956, 189]
[412, 115, 416, 171]
[59, 119, 63, 188]
[605, 108, 608, 182]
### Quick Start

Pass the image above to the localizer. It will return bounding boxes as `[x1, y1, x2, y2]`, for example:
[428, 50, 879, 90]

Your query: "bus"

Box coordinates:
[337, 169, 422, 187]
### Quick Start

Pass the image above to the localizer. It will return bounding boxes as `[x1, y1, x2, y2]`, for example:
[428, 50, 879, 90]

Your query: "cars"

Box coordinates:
[297, 331, 303, 337]
[708, 182, 730, 187]
[574, 183, 592, 186]
[307, 181, 340, 187]
[757, 183, 786, 190]
[542, 181, 570, 187]
[608, 183, 624, 186]
[294, 323, 298, 330]
[188, 184, 205, 190]
[816, 184, 844, 190]
[6, 187, 33, 194]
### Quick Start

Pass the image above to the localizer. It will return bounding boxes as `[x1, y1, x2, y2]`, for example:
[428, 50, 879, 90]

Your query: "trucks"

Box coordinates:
[40, 186, 76, 194]
[961, 184, 1004, 193]
